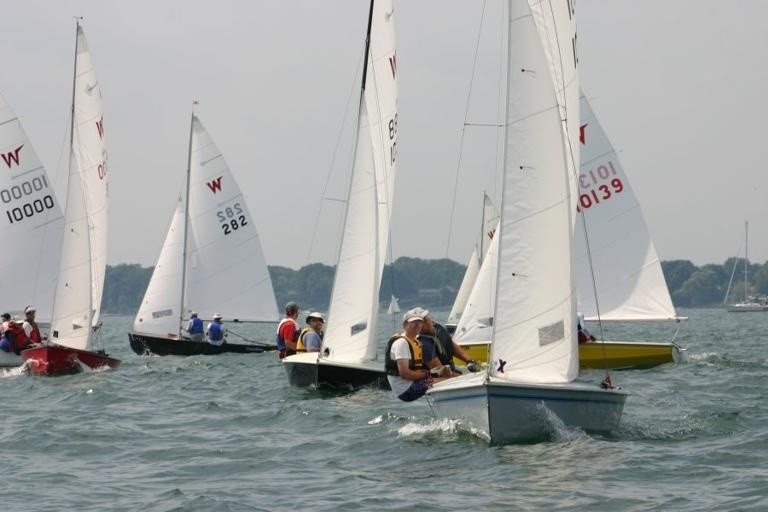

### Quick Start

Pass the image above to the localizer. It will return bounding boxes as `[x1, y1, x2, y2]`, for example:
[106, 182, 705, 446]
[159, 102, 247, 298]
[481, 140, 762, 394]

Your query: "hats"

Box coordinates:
[285, 301, 301, 312]
[24, 307, 37, 315]
[410, 306, 431, 319]
[0, 313, 11, 319]
[402, 312, 424, 322]
[191, 311, 197, 317]
[306, 312, 325, 324]
[212, 313, 223, 319]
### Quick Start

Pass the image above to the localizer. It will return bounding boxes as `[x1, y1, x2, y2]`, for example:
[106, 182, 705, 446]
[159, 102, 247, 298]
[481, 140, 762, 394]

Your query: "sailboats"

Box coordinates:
[723, 221, 767, 312]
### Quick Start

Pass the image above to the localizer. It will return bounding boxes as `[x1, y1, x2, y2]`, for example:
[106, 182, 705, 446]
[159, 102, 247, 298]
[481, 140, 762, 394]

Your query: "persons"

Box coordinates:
[385, 310, 450, 402]
[5, 320, 33, 355]
[277, 302, 301, 359]
[22, 304, 42, 345]
[205, 313, 228, 346]
[181, 311, 204, 342]
[0, 314, 11, 337]
[411, 307, 485, 378]
[296, 312, 325, 355]
